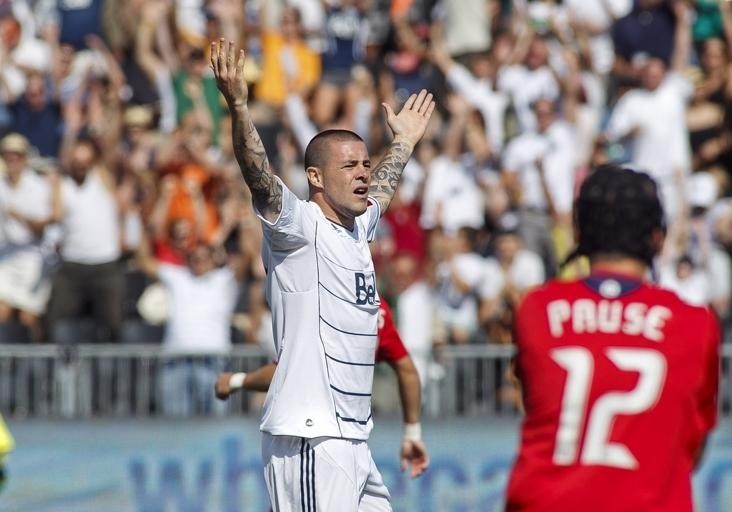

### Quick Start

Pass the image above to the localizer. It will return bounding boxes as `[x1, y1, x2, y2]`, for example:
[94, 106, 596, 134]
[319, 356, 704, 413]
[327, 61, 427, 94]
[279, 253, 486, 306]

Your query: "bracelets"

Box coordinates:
[401, 421, 421, 441]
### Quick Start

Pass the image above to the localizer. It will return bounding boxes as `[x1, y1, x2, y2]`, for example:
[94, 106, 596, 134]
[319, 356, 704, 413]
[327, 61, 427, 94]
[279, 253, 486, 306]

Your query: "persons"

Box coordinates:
[210, 38, 435, 512]
[215, 290, 430, 480]
[1, 1, 732, 421]
[503, 165, 721, 512]
[0, 414, 16, 492]
